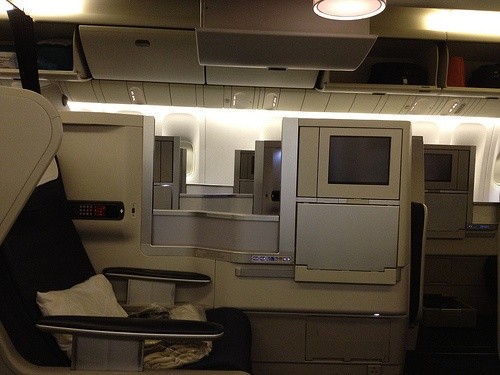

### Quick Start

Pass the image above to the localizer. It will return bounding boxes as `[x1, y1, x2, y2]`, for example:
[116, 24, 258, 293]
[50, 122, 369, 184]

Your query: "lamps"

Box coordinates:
[312, 0, 388, 21]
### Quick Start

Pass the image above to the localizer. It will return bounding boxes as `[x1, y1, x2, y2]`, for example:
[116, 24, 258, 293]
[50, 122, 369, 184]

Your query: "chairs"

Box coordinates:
[0, 153, 252, 374]
